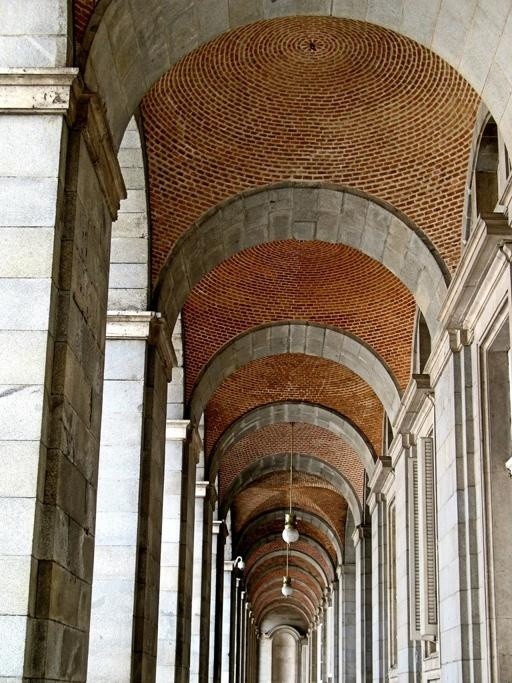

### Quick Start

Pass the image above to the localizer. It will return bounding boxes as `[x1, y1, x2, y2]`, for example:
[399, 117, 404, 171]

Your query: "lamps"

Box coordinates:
[282, 421, 299, 544]
[281, 546, 294, 596]
